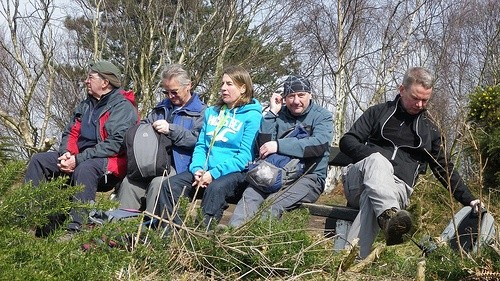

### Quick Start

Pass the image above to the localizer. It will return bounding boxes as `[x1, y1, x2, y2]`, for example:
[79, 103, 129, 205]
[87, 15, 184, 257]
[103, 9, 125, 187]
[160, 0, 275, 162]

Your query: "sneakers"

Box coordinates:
[377, 207, 412, 247]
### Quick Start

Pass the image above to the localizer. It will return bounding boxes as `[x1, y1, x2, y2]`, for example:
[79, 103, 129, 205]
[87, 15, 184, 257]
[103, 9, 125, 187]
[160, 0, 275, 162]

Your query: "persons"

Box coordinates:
[118, 63, 208, 218]
[215, 74, 333, 234]
[339, 66, 485, 258]
[20, 61, 141, 231]
[161, 65, 263, 230]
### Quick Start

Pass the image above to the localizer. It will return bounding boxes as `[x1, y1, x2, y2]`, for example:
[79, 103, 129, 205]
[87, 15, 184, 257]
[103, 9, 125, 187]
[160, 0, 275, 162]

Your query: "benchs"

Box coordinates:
[188, 145, 360, 254]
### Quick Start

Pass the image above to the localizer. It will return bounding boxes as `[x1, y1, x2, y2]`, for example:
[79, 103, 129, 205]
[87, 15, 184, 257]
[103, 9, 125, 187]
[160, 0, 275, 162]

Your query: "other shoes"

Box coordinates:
[81, 236, 123, 252]
[57, 230, 77, 242]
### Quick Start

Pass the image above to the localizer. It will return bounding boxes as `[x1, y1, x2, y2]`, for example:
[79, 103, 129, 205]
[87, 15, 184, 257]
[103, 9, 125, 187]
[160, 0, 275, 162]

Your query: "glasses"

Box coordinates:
[87, 74, 101, 82]
[163, 86, 182, 96]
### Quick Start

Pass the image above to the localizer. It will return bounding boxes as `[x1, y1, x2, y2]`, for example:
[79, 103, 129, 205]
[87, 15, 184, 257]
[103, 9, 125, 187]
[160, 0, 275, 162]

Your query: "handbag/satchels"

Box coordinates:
[88, 209, 158, 226]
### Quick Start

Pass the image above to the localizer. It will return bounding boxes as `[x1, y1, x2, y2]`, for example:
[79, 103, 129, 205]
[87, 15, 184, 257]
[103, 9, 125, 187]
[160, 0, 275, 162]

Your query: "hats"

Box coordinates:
[89, 62, 122, 88]
[281, 75, 312, 98]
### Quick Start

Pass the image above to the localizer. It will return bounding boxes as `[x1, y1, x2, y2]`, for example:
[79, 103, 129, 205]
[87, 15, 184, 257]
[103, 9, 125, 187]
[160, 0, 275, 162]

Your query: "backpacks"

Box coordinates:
[245, 123, 309, 193]
[422, 202, 495, 257]
[124, 118, 172, 181]
